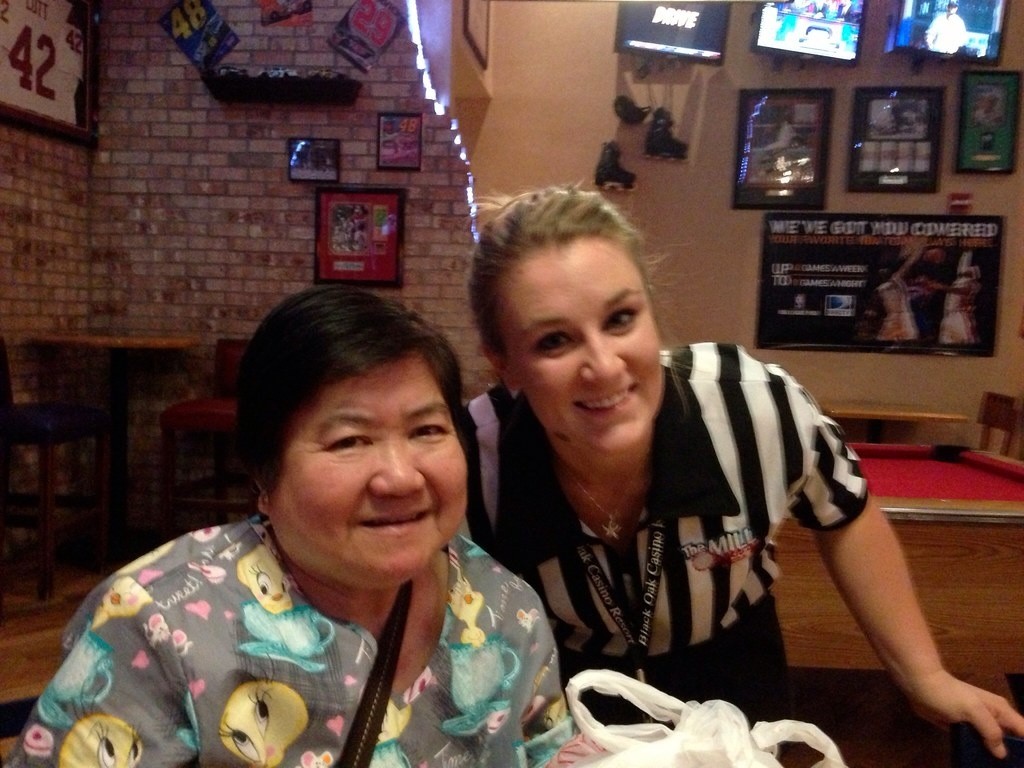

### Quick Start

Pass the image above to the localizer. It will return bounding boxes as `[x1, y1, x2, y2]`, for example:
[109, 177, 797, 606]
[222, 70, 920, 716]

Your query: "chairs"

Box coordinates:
[0, 337, 110, 600]
[157, 336, 256, 543]
[978, 391, 1024, 461]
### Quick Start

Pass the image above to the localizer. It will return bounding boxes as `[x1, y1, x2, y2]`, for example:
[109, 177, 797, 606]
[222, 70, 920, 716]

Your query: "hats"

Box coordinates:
[948, 0, 958, 7]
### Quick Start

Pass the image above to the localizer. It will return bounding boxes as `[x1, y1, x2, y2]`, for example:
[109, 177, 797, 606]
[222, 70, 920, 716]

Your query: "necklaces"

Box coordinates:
[560, 451, 646, 540]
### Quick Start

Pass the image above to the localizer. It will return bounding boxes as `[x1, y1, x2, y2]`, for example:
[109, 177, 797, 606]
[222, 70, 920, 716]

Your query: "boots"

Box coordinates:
[594, 141, 637, 192]
[641, 106, 689, 163]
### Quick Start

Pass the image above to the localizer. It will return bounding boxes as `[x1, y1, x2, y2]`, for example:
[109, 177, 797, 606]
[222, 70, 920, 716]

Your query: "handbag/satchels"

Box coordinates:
[565, 669, 848, 768]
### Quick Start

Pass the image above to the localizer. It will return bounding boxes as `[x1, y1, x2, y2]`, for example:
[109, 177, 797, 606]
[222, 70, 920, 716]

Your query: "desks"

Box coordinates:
[34, 334, 205, 570]
[819, 400, 971, 444]
[771, 443, 1024, 712]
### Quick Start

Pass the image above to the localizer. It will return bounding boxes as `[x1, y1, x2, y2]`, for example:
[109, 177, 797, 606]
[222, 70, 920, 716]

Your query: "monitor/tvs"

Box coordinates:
[613, 1, 730, 65]
[894, 0, 1009, 67]
[750, 0, 868, 67]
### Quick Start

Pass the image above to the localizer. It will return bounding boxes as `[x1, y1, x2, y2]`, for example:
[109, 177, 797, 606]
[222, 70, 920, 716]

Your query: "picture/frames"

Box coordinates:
[954, 70, 1021, 175]
[463, 0, 491, 71]
[376, 111, 423, 172]
[0, 0, 95, 146]
[286, 135, 341, 183]
[729, 88, 834, 210]
[845, 84, 945, 194]
[313, 184, 407, 290]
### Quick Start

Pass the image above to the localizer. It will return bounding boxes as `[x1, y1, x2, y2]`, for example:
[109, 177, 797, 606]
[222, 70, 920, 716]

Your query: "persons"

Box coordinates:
[459, 188, 1024, 760]
[0, 288, 576, 768]
[925, 2, 967, 54]
[876, 247, 984, 345]
